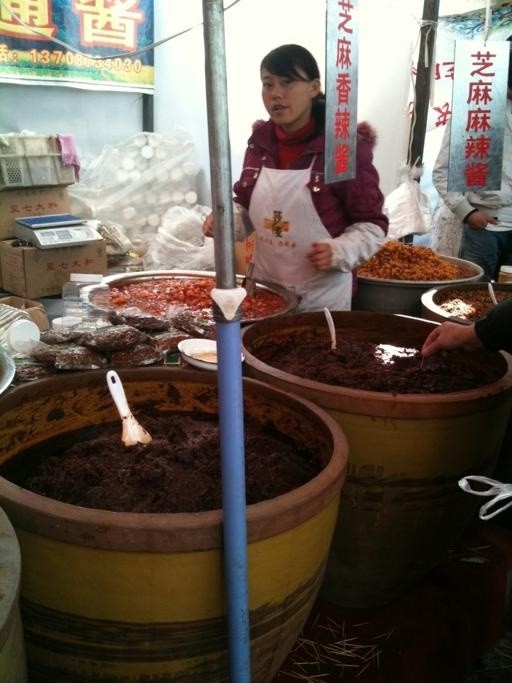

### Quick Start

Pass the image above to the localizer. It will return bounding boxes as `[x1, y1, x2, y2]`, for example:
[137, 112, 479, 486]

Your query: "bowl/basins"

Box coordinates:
[81, 270, 300, 334]
[357, 252, 485, 315]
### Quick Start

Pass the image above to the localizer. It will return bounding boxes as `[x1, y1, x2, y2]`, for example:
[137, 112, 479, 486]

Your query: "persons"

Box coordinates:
[431, 32, 511, 283]
[420, 295, 512, 360]
[200, 42, 390, 312]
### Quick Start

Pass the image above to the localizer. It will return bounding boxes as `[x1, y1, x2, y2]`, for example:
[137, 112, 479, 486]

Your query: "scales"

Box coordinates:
[16, 212, 103, 250]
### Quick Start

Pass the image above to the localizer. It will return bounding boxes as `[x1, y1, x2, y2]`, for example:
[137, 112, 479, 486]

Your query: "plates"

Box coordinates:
[175, 337, 244, 373]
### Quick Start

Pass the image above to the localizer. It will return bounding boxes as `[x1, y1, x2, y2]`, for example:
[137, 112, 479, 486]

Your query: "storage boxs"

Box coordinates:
[0, 239, 108, 300]
[0, 189, 71, 238]
[0, 296, 49, 332]
